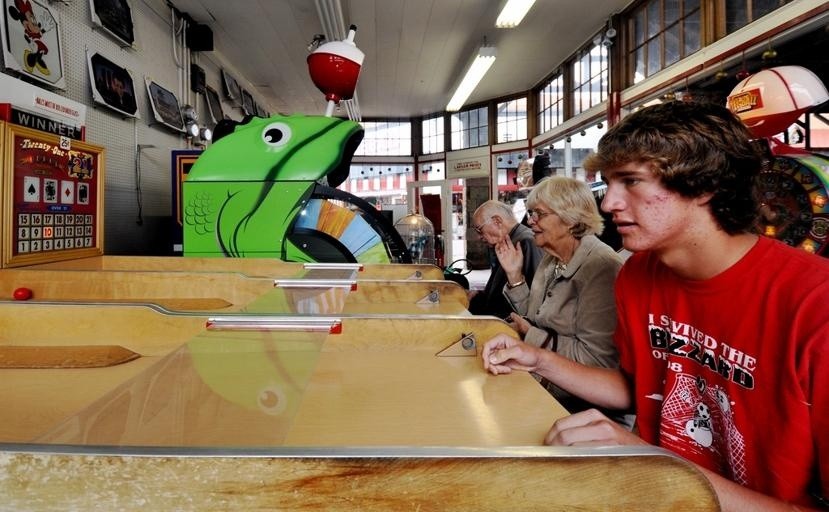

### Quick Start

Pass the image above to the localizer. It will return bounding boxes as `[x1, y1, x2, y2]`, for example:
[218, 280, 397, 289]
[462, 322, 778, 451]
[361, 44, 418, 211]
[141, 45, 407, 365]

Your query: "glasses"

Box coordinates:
[528, 210, 558, 220]
[474, 217, 493, 234]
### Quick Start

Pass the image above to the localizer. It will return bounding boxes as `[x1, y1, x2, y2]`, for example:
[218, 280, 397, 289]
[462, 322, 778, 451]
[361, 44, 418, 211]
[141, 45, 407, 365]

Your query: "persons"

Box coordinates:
[495, 176, 626, 398]
[483, 101, 829, 512]
[464, 200, 543, 319]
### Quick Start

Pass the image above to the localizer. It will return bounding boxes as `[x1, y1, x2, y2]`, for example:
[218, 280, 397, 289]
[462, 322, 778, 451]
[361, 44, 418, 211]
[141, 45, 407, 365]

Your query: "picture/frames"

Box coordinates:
[0, 119, 107, 269]
[241, 89, 255, 116]
[144, 75, 187, 133]
[0, 0, 67, 93]
[89, 0, 136, 48]
[85, 44, 141, 121]
[204, 84, 224, 125]
[220, 67, 243, 108]
[256, 103, 268, 118]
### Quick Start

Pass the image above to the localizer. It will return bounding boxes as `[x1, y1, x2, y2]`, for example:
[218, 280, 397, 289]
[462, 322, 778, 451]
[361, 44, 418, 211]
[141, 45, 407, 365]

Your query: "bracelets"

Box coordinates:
[509, 276, 525, 287]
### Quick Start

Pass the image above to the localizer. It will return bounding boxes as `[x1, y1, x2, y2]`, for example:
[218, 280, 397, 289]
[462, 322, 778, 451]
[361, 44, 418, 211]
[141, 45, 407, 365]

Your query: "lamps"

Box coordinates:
[443, 34, 498, 112]
[187, 124, 199, 137]
[493, 0, 535, 30]
[200, 128, 212, 141]
